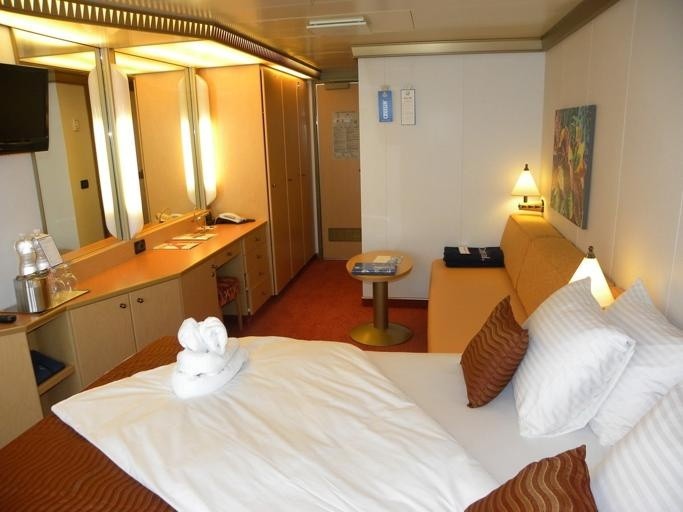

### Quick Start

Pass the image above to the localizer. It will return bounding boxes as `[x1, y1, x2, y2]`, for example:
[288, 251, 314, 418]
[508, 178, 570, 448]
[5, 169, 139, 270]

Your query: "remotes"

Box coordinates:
[0, 314, 17, 323]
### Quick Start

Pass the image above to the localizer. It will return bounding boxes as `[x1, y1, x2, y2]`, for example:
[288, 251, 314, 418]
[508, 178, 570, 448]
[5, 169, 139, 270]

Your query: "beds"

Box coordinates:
[0, 281, 681, 512]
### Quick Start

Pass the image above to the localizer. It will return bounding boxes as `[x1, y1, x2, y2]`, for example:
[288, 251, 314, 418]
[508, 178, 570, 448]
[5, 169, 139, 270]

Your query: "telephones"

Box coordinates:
[216, 212, 256, 224]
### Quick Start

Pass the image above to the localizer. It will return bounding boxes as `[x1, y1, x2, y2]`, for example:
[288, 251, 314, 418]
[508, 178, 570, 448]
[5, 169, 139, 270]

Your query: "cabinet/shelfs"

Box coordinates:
[180, 261, 220, 333]
[37, 279, 182, 421]
[129, 69, 201, 224]
[196, 62, 314, 295]
[0, 310, 81, 454]
[217, 226, 271, 318]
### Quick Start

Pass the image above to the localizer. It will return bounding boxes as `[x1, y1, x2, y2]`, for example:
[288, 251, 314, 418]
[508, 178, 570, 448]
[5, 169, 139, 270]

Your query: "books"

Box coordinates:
[351, 263, 397, 276]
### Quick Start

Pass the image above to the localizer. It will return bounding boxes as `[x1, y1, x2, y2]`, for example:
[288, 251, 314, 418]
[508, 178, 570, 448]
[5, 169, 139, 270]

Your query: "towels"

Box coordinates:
[166, 312, 249, 399]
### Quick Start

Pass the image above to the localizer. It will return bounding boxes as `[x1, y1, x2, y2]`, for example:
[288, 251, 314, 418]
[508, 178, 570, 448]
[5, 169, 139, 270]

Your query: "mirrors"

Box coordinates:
[0, 22, 122, 280]
[102, 46, 203, 237]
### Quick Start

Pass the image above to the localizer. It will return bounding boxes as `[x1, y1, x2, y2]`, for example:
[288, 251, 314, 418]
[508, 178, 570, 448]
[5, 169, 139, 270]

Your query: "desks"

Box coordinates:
[344, 250, 417, 347]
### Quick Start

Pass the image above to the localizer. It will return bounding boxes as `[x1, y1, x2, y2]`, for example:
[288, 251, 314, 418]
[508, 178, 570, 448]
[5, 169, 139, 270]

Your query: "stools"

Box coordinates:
[216, 275, 244, 331]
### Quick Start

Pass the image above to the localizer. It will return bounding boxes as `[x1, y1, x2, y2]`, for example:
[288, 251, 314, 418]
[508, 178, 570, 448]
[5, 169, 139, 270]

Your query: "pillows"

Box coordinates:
[510, 275, 633, 441]
[458, 294, 530, 408]
[456, 437, 598, 512]
[595, 377, 682, 512]
[587, 276, 681, 443]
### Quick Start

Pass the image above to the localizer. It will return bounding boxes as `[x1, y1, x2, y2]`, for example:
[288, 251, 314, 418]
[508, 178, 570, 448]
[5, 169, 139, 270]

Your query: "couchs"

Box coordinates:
[427, 211, 620, 354]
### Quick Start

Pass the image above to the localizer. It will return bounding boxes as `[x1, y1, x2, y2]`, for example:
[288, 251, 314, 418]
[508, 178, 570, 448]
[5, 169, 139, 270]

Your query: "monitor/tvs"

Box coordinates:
[0, 62, 49, 153]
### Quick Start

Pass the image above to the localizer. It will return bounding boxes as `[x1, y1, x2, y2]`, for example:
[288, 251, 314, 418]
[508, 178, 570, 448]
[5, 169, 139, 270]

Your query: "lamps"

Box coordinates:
[510, 163, 544, 214]
[565, 244, 614, 309]
